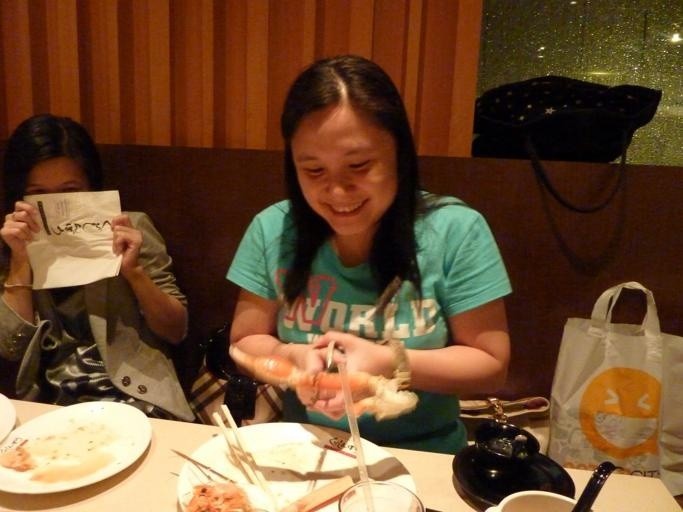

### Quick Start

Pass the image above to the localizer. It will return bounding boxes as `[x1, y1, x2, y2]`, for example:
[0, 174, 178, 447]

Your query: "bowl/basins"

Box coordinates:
[492, 491, 590, 512]
[477, 422, 541, 476]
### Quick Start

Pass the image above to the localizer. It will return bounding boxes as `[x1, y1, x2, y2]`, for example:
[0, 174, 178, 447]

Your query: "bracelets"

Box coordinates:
[386, 339, 412, 391]
[3, 281, 32, 289]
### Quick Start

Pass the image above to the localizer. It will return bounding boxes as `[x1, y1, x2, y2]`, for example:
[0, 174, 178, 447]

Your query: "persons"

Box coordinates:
[0, 114, 196, 422]
[225, 55, 514, 455]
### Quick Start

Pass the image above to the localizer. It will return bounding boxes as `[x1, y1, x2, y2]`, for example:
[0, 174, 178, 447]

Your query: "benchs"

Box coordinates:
[95, 144, 683, 400]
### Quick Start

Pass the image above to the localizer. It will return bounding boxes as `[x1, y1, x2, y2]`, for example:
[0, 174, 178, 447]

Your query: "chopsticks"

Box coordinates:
[209, 403, 291, 512]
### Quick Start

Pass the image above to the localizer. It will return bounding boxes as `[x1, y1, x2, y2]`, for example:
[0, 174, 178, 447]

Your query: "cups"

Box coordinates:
[337, 482, 424, 512]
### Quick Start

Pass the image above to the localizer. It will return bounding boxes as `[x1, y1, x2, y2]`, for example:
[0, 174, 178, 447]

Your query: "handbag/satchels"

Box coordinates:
[471, 75, 662, 212]
[189, 321, 283, 427]
[459, 396, 551, 441]
[546, 281, 683, 477]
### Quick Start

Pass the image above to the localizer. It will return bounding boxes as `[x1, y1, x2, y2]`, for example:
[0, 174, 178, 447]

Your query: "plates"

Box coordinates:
[176, 423, 417, 512]
[0, 392, 15, 449]
[452, 441, 576, 511]
[0, 401, 152, 495]
